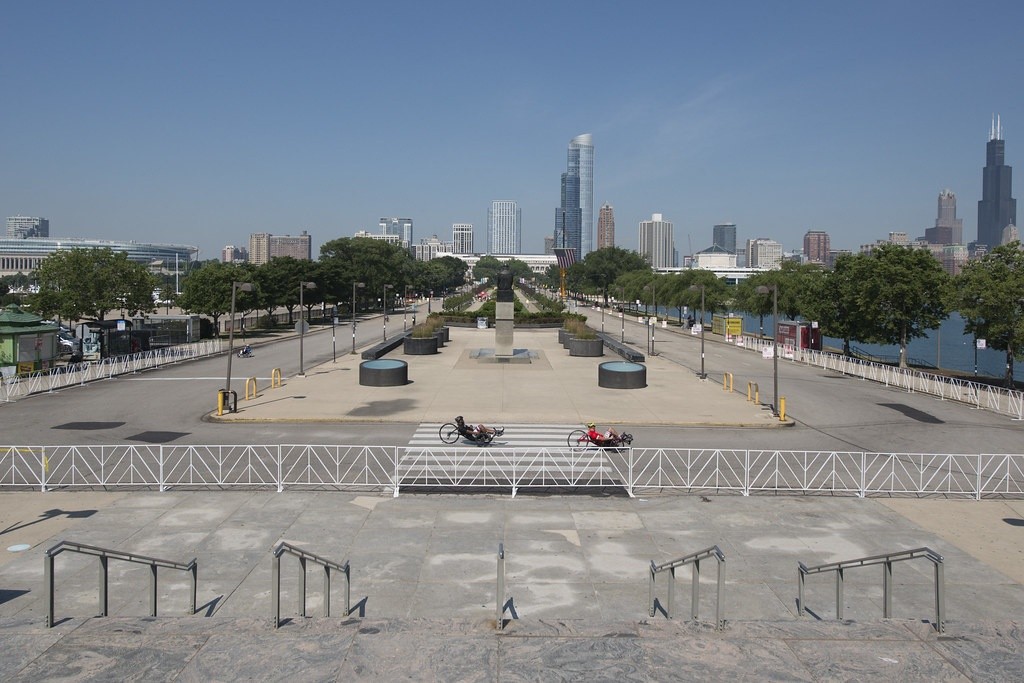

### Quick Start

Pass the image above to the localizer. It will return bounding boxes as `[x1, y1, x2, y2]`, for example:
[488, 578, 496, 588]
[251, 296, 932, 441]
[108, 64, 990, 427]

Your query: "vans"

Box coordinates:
[40, 319, 76, 355]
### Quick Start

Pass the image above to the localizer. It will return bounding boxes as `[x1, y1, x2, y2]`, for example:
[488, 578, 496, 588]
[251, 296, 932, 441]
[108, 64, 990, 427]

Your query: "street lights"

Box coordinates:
[223, 281, 256, 410]
[296, 281, 317, 377]
[383, 284, 394, 343]
[351, 282, 366, 354]
[403, 285, 414, 332]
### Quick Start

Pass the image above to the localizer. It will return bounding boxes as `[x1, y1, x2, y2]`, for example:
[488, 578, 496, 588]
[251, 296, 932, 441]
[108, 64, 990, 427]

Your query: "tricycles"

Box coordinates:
[236, 348, 253, 358]
[567, 429, 633, 454]
[439, 422, 505, 447]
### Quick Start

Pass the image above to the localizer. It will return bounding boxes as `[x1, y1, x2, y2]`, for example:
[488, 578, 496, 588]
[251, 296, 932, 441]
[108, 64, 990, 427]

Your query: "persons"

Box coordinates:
[688, 315, 693, 326]
[455, 415, 504, 441]
[242, 343, 251, 354]
[595, 302, 599, 307]
[588, 421, 625, 447]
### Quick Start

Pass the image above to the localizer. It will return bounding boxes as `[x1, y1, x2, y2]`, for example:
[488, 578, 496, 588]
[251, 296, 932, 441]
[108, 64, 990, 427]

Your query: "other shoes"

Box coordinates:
[499, 428, 504, 434]
[484, 437, 493, 442]
[619, 432, 625, 439]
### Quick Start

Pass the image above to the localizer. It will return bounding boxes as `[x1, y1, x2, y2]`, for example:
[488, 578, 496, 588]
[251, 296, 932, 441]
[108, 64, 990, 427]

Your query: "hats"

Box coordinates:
[455, 416, 463, 422]
[588, 422, 595, 428]
